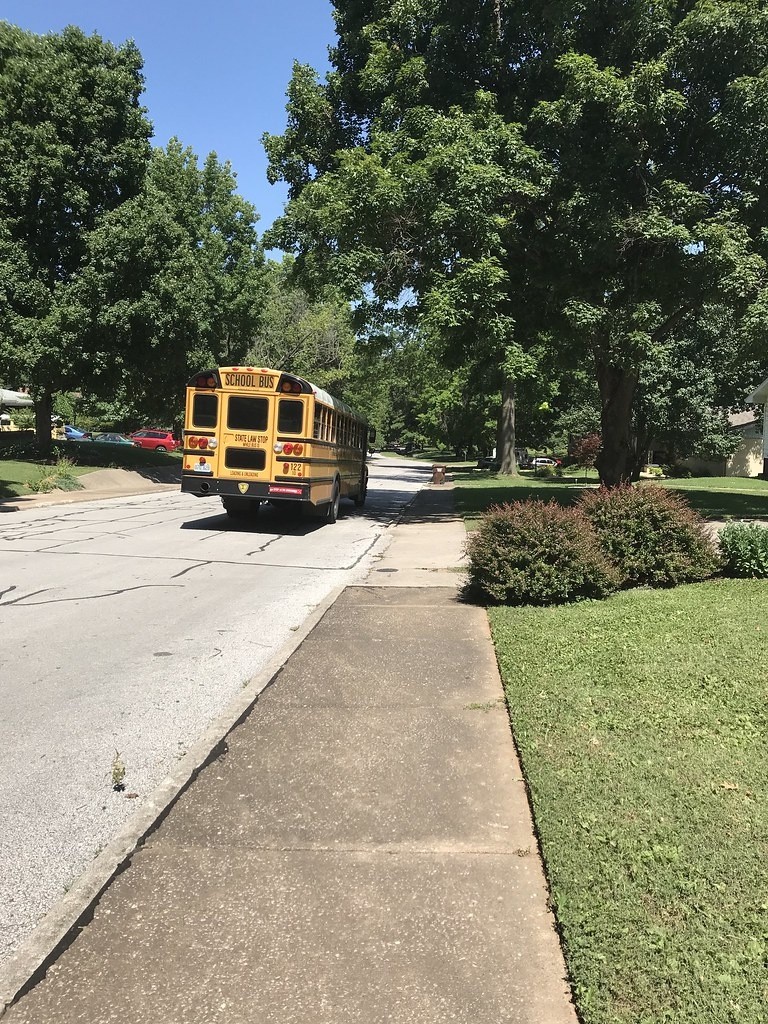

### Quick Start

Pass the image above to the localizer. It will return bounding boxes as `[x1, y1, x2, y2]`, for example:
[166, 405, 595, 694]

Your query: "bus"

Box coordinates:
[181, 366, 377, 523]
[0, 413, 67, 442]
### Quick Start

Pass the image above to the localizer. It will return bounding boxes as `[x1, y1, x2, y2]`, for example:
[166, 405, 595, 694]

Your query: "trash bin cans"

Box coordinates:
[432, 464, 446, 484]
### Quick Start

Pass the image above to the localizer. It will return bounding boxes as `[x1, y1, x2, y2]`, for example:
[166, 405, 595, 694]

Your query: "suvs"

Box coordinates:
[128, 429, 177, 452]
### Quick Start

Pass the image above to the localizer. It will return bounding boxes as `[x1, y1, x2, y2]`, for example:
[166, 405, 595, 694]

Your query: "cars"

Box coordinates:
[69, 433, 136, 448]
[476, 457, 557, 471]
[62, 424, 93, 442]
